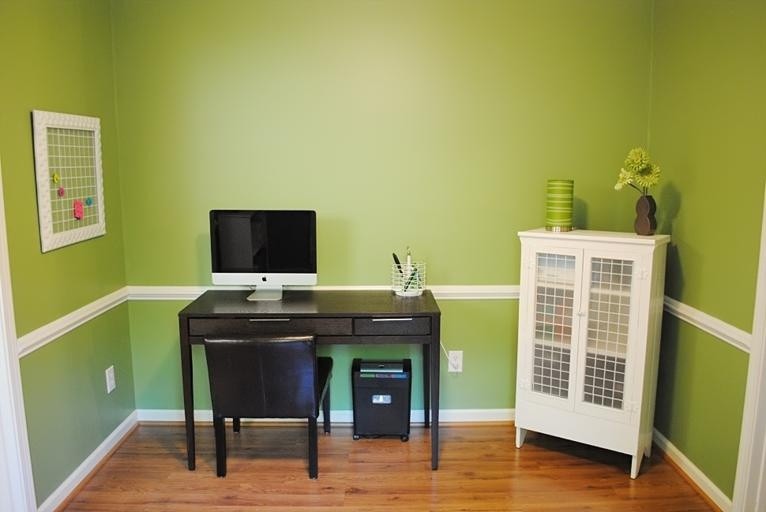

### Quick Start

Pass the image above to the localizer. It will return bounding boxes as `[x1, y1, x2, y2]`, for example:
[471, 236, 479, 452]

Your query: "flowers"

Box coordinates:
[614, 146, 662, 198]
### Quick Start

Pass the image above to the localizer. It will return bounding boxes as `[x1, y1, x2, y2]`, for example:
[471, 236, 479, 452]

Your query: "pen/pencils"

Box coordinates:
[393, 246, 421, 292]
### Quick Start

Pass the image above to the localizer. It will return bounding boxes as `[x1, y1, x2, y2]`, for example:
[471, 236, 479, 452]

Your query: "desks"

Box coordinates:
[178, 288, 440, 470]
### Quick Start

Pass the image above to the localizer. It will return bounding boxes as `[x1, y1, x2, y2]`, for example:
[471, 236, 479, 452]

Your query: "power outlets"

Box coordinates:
[448, 350, 461, 373]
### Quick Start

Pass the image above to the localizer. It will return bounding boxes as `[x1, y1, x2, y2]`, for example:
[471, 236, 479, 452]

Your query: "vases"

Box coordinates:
[633, 195, 657, 235]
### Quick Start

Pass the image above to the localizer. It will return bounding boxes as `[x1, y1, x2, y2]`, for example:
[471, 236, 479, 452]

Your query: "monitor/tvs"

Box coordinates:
[209, 209, 319, 301]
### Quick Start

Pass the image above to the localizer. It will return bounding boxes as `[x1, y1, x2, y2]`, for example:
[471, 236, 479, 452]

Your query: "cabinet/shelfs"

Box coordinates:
[514, 226, 672, 477]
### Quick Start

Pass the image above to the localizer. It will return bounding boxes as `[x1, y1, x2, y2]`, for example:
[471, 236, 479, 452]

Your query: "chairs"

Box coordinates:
[202, 336, 333, 478]
